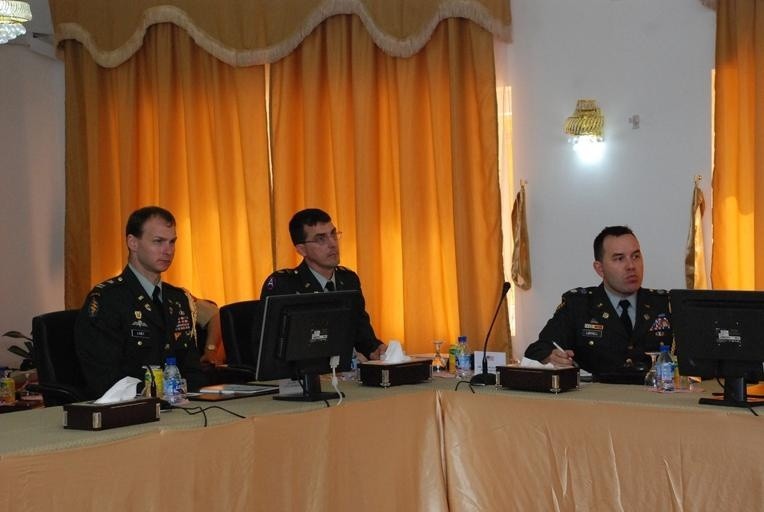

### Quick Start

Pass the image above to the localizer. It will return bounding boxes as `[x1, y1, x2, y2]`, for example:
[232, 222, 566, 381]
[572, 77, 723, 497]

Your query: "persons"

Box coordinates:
[71, 206, 202, 400]
[523, 225, 677, 376]
[259, 208, 389, 361]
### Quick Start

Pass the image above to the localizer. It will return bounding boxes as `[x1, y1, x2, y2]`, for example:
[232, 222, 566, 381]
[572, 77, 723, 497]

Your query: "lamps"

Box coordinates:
[0, 0, 33, 47]
[563, 98, 606, 167]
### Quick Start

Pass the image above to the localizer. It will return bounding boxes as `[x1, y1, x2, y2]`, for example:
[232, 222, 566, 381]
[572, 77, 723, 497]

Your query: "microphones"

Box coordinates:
[470, 282, 510, 385]
[90, 320, 171, 410]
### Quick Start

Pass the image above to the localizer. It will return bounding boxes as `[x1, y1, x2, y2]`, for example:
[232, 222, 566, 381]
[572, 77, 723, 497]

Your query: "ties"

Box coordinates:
[153, 286, 164, 316]
[325, 281, 334, 291]
[619, 300, 632, 336]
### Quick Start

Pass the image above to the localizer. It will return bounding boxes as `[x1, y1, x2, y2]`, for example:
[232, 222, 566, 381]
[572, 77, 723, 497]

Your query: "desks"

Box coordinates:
[437, 357, 764, 512]
[0, 362, 444, 512]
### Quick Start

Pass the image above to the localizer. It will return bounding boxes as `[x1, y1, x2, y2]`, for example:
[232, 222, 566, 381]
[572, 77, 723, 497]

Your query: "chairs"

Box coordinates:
[217, 299, 268, 380]
[30, 308, 92, 410]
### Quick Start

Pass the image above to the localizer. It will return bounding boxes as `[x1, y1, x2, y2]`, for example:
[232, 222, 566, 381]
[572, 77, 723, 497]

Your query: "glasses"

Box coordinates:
[295, 232, 342, 244]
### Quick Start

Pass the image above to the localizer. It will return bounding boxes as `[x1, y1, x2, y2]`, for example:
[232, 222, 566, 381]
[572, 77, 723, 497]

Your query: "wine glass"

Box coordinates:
[643, 350, 665, 394]
[141, 365, 161, 370]
[428, 339, 448, 380]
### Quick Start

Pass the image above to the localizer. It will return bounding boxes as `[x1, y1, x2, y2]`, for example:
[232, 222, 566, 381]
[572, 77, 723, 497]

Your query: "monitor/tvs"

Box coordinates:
[670, 289, 764, 407]
[256, 290, 360, 401]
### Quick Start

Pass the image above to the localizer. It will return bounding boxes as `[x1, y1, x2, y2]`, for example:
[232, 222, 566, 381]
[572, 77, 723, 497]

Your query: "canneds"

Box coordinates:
[145, 369, 163, 396]
[449, 345, 456, 374]
[674, 363, 688, 389]
[0, 378, 16, 403]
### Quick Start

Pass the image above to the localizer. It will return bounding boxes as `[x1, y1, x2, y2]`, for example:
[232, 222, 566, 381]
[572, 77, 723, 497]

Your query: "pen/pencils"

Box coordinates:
[200, 390, 235, 394]
[552, 341, 579, 368]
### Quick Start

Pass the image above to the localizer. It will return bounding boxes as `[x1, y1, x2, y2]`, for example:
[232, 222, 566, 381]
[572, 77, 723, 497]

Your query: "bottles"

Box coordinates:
[653, 348, 677, 393]
[163, 357, 183, 405]
[453, 336, 472, 381]
